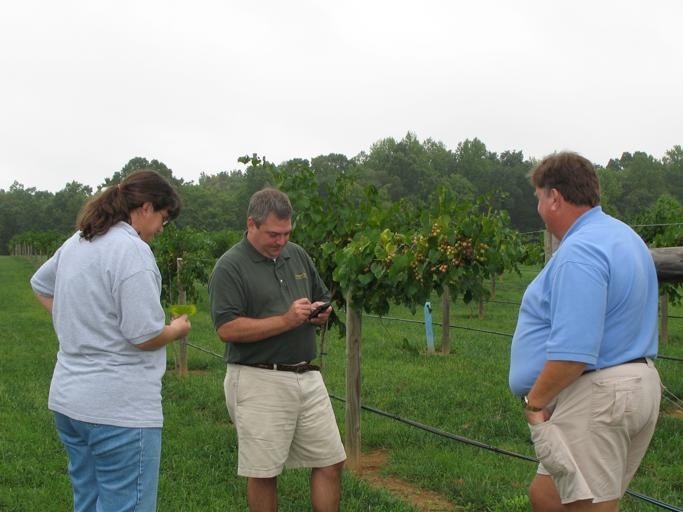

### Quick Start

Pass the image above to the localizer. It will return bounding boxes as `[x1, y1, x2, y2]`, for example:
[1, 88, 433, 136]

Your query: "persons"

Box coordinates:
[508, 150, 663, 512]
[28, 168, 193, 512]
[206, 186, 350, 511]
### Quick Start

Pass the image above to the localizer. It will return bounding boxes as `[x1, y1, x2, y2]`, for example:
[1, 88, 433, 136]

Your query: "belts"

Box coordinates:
[583, 357, 646, 374]
[244, 362, 320, 371]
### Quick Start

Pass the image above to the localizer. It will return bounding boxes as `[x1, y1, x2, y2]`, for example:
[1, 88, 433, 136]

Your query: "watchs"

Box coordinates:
[520, 396, 542, 413]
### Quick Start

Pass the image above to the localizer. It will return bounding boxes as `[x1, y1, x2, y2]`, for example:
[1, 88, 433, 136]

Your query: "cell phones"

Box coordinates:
[308, 301, 330, 320]
